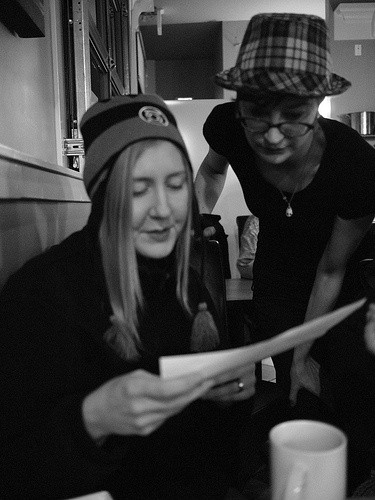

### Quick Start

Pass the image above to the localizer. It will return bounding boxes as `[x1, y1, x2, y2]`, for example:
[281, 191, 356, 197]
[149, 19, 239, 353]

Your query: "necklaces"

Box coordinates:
[273, 151, 308, 218]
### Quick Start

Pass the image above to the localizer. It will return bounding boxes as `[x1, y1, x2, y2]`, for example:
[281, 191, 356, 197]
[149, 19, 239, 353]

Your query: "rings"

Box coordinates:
[238, 380, 243, 391]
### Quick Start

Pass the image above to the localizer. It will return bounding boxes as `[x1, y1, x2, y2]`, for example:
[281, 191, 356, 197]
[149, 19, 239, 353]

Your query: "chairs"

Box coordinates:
[202, 214, 249, 279]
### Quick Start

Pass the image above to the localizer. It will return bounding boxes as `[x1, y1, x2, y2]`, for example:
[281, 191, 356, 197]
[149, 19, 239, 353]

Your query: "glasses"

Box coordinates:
[235, 97, 319, 139]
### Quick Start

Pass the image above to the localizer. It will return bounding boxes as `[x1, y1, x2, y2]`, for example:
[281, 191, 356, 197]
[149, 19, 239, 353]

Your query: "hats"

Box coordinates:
[214, 12, 352, 96]
[78, 93, 192, 193]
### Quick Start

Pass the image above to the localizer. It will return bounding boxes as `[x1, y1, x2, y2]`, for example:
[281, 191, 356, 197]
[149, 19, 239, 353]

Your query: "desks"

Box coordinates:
[226, 279, 253, 302]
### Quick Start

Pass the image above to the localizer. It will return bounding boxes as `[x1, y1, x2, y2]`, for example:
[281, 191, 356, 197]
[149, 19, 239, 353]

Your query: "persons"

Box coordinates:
[193, 11, 375, 500]
[0, 93, 259, 500]
[236, 213, 261, 279]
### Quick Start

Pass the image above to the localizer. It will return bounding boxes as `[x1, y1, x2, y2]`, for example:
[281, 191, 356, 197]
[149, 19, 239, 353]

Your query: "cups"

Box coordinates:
[269, 418, 346, 500]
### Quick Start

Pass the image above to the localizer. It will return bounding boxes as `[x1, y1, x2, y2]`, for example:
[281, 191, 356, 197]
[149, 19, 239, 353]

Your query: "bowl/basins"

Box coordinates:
[345, 112, 375, 135]
